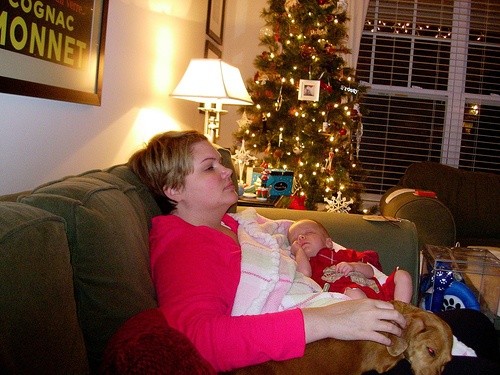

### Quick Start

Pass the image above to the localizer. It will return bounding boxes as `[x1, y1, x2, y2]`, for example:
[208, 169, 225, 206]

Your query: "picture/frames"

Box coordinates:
[0, 0, 110, 107]
[298, 79, 320, 102]
[206, 0, 226, 45]
[199, 39, 223, 118]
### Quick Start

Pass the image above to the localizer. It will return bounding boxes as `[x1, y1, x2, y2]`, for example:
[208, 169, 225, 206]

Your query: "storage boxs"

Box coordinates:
[251, 168, 294, 196]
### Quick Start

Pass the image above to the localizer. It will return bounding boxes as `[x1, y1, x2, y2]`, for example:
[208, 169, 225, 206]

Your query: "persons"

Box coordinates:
[131, 130, 500, 375]
[287, 219, 413, 304]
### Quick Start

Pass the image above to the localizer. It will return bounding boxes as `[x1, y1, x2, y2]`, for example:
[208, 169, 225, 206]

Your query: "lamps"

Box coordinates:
[169, 58, 256, 144]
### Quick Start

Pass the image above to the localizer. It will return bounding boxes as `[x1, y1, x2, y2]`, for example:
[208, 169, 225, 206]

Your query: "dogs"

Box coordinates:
[212, 300, 453, 375]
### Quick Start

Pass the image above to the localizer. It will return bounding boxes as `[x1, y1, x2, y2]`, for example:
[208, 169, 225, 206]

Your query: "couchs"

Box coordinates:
[0, 143, 419, 375]
[379, 161, 500, 251]
